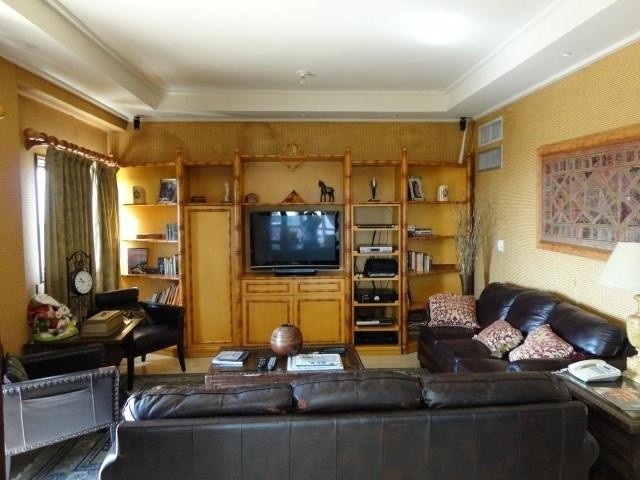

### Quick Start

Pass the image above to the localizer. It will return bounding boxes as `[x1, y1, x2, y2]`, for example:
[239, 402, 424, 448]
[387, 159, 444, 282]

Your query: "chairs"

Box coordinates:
[0, 284, 187, 480]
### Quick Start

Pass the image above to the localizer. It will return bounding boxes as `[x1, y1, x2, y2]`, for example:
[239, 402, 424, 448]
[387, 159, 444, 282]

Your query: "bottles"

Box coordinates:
[133, 186, 146, 204]
[436, 185, 449, 201]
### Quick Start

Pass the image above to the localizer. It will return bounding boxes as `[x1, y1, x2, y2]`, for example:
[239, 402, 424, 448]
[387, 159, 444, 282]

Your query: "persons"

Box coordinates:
[162, 182, 173, 200]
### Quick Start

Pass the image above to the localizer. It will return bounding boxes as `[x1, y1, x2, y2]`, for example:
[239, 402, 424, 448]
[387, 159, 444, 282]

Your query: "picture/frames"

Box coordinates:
[533, 123, 640, 264]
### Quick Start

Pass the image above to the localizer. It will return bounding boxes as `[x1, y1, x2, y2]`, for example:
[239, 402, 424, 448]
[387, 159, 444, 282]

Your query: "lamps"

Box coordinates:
[601, 242, 640, 385]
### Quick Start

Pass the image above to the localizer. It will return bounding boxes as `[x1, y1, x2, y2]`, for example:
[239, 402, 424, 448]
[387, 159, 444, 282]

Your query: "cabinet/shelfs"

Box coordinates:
[241, 278, 346, 348]
[348, 161, 403, 357]
[183, 156, 240, 358]
[241, 151, 346, 208]
[406, 160, 475, 355]
[112, 160, 182, 358]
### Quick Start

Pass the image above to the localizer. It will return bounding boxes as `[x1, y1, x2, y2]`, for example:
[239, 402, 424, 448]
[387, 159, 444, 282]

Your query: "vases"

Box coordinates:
[460, 275, 473, 296]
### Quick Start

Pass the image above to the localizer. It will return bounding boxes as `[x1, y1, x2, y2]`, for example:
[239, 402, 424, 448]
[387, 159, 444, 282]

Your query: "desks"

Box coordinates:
[561, 361, 640, 480]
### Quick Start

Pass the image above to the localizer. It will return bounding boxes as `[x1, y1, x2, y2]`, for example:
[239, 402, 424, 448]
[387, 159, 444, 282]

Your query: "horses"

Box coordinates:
[318, 178, 335, 203]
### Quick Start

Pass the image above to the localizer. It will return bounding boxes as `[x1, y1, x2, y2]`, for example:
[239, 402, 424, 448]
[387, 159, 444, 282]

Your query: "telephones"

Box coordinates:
[568, 359, 623, 383]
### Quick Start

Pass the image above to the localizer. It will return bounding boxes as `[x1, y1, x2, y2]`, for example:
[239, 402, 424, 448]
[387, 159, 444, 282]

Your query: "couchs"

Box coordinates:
[418, 277, 631, 374]
[96, 367, 599, 479]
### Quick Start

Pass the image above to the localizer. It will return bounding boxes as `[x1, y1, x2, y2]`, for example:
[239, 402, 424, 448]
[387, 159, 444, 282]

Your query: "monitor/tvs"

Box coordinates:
[244, 203, 345, 276]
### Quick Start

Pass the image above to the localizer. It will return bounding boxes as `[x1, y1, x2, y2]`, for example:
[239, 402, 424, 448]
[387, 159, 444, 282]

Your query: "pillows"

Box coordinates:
[425, 292, 578, 363]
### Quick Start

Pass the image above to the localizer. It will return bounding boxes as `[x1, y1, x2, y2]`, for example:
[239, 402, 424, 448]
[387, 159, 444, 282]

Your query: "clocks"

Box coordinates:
[70, 269, 94, 296]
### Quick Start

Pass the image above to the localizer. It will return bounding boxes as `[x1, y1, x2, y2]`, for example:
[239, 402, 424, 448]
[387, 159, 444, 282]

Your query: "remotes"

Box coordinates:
[256, 356, 266, 367]
[267, 356, 276, 370]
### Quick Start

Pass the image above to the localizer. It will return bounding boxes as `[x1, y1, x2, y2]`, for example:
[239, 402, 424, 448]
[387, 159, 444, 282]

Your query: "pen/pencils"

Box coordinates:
[312, 352, 345, 354]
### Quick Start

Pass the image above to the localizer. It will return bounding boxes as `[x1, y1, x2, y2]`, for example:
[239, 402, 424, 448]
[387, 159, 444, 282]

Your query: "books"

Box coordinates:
[80, 310, 145, 345]
[163, 252, 179, 278]
[156, 284, 180, 307]
[286, 353, 345, 373]
[165, 222, 178, 240]
[592, 386, 640, 419]
[212, 350, 250, 366]
[407, 251, 433, 274]
